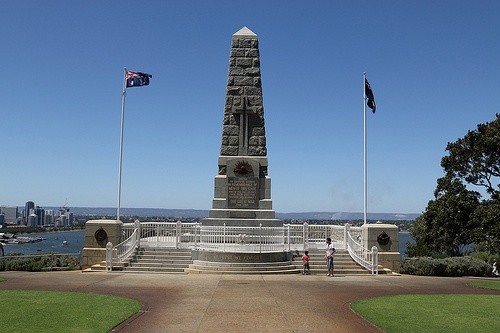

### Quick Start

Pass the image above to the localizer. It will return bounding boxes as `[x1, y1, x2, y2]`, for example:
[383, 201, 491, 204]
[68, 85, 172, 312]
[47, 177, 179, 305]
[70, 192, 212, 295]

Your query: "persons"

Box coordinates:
[0, 243, 4, 257]
[324, 238, 336, 277]
[301, 251, 311, 275]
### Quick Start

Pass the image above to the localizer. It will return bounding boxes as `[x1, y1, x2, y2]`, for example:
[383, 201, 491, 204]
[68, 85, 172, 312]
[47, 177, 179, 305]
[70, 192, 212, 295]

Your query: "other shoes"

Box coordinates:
[302, 272, 305, 275]
[330, 274, 334, 277]
[309, 272, 311, 275]
[327, 273, 331, 276]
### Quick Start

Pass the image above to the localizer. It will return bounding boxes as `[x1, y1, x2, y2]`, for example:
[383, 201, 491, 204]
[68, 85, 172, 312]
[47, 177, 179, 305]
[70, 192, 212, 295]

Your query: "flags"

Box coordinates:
[365, 78, 376, 114]
[126, 71, 153, 88]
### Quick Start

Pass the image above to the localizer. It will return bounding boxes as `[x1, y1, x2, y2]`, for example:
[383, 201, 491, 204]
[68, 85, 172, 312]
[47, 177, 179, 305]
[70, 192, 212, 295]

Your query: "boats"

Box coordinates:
[61, 241, 68, 247]
